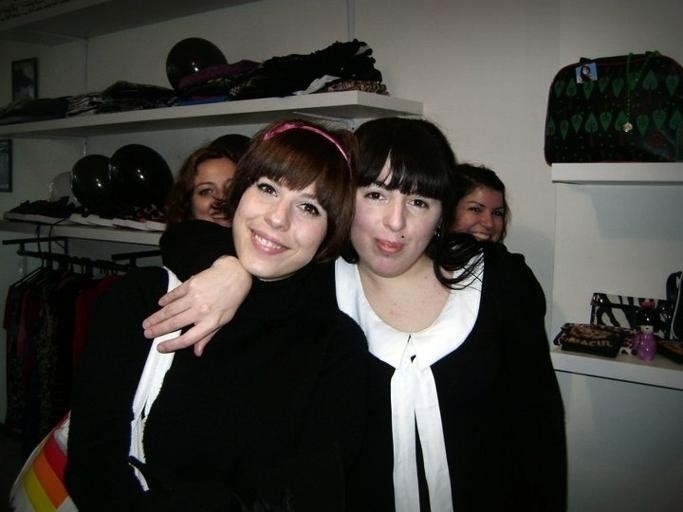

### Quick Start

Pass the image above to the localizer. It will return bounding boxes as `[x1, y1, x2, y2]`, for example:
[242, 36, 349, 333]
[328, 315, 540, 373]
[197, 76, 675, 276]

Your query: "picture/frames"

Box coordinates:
[11, 56, 38, 103]
[0, 139, 13, 193]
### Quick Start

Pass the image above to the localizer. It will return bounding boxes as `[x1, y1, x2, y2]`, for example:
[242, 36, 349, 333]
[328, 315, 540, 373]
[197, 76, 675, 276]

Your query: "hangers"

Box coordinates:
[10, 252, 118, 295]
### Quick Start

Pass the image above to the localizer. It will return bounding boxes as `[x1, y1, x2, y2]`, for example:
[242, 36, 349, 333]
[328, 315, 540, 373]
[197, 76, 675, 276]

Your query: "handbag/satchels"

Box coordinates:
[590, 292, 669, 340]
[554, 322, 640, 357]
[10, 266, 185, 512]
[545, 50, 683, 166]
[562, 325, 623, 358]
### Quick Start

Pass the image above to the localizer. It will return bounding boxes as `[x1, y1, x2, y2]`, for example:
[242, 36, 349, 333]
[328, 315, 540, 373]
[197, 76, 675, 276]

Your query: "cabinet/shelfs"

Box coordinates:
[550, 164, 683, 512]
[0, 91, 424, 246]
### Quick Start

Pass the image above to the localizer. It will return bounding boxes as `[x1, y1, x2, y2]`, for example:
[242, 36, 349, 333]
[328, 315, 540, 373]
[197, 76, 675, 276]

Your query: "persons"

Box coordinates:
[444, 164, 508, 242]
[143, 116, 568, 512]
[63, 115, 356, 510]
[175, 139, 234, 227]
